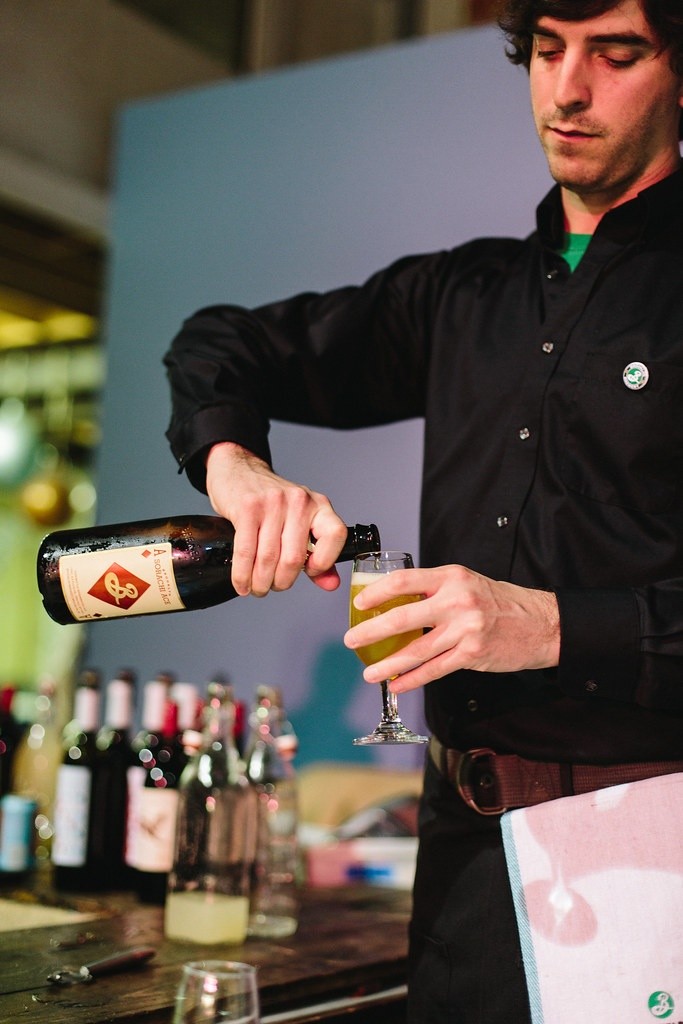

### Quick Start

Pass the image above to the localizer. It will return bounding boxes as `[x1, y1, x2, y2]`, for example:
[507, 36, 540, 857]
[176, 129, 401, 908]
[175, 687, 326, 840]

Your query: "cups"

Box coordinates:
[174, 961, 260, 1024]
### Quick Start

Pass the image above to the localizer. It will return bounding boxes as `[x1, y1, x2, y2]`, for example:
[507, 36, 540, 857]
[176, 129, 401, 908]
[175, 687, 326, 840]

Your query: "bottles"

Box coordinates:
[0, 669, 303, 944]
[37, 515, 379, 623]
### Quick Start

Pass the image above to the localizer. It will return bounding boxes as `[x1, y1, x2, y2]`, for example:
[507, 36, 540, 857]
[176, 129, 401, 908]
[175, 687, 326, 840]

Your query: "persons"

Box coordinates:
[164, 0, 683, 1024]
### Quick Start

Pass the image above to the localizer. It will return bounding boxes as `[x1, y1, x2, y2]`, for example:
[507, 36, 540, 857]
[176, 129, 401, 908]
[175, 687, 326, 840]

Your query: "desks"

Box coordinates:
[0, 879, 422, 1024]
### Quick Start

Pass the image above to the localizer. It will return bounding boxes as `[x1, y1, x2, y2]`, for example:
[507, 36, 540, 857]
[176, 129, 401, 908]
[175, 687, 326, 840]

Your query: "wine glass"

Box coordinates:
[349, 552, 429, 746]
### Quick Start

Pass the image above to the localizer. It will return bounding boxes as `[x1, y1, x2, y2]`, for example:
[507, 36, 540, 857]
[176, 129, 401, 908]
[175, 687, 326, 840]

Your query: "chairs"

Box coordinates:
[502, 773, 683, 1024]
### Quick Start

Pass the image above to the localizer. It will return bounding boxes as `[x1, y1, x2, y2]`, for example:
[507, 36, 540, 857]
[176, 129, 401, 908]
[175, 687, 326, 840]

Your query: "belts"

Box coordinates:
[427, 734, 683, 815]
